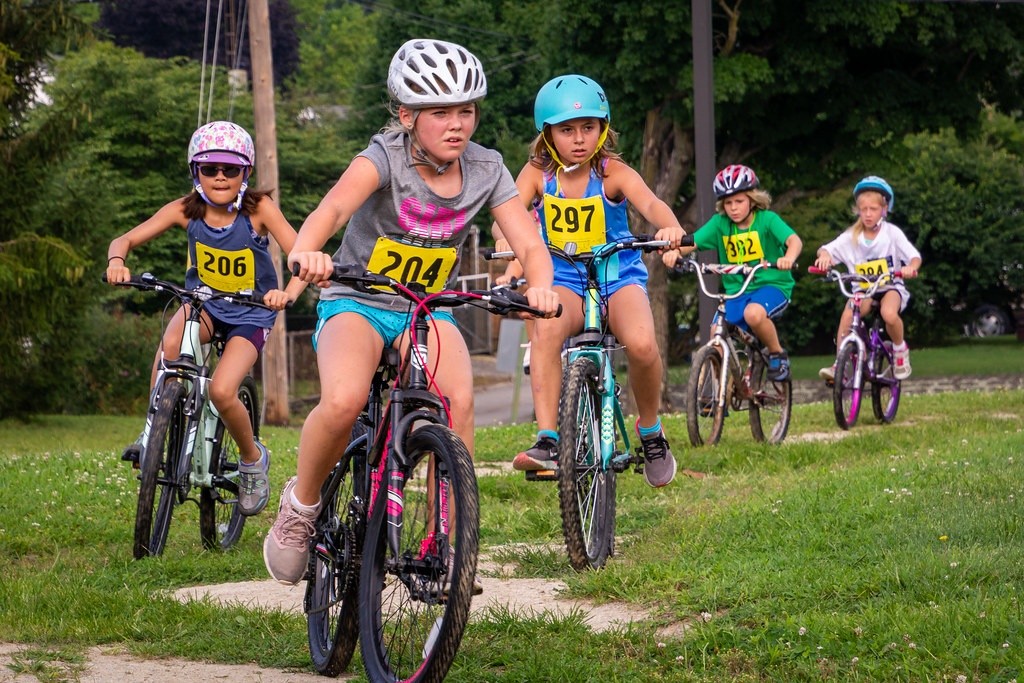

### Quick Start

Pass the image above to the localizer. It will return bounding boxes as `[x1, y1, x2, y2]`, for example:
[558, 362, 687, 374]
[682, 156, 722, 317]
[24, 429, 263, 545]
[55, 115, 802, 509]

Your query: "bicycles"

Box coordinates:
[291, 258, 568, 683]
[482, 231, 696, 572]
[101, 269, 294, 562]
[809, 265, 920, 430]
[673, 258, 800, 449]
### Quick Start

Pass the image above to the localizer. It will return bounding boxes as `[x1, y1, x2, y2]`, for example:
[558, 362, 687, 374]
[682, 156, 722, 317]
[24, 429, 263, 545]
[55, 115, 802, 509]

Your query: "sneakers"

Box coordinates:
[513, 434, 560, 470]
[410, 545, 484, 597]
[121, 446, 142, 461]
[700, 402, 729, 417]
[635, 418, 677, 489]
[893, 343, 913, 380]
[263, 475, 323, 586]
[766, 349, 791, 382]
[819, 363, 849, 386]
[237, 441, 270, 517]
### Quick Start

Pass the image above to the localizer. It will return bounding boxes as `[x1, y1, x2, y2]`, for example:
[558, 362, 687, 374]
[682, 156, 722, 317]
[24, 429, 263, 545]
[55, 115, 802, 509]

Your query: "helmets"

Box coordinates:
[853, 175, 894, 213]
[187, 121, 255, 179]
[712, 164, 759, 202]
[388, 39, 488, 110]
[534, 74, 611, 134]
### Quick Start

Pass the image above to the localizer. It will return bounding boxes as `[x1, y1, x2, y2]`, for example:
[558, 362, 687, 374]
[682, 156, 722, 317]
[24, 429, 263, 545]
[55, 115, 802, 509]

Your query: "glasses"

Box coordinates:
[197, 164, 245, 178]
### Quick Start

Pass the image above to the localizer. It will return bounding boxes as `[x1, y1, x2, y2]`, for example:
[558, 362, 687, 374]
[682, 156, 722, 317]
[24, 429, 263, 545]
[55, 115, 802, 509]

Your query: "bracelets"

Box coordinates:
[677, 247, 681, 254]
[108, 256, 125, 264]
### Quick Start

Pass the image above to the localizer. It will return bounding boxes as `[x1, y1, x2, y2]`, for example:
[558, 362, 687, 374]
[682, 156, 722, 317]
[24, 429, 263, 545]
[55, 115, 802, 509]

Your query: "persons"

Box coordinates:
[491, 75, 687, 487]
[106, 121, 308, 517]
[814, 176, 921, 379]
[263, 38, 560, 585]
[662, 165, 803, 400]
